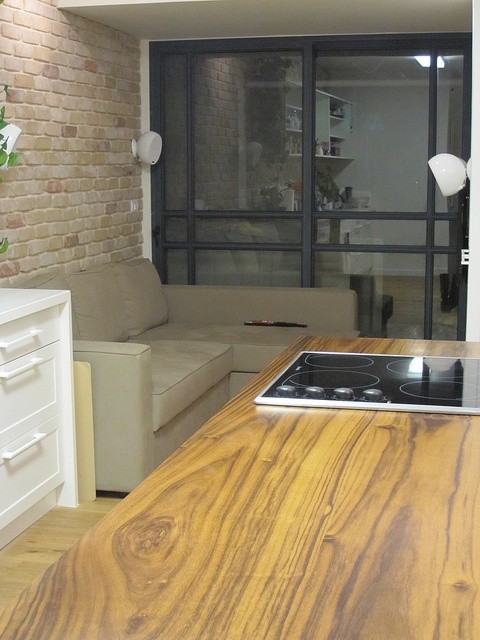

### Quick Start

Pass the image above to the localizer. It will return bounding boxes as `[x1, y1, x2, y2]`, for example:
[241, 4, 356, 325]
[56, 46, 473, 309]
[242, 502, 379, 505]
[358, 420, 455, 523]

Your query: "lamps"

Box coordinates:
[131, 131, 162, 165]
[412, 55, 445, 69]
[0, 123, 22, 171]
[426, 153, 471, 199]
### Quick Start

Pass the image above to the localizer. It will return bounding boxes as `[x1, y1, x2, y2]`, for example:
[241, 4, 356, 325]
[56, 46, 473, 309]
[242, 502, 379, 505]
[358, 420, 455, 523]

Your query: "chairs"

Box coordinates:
[333, 274, 394, 338]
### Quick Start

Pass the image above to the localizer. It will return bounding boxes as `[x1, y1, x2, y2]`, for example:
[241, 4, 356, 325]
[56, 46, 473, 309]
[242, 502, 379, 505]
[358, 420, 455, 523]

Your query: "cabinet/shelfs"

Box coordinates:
[241, 75, 355, 161]
[275, 187, 375, 244]
[0, 288, 78, 553]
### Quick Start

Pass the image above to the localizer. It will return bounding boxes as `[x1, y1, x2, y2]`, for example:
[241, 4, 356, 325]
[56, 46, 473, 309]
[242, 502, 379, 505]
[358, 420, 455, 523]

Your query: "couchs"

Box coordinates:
[166, 216, 351, 289]
[21, 256, 360, 494]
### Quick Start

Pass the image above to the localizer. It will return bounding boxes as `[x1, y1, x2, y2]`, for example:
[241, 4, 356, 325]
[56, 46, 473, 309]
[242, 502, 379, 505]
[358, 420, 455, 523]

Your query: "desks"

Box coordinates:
[3, 333, 480, 639]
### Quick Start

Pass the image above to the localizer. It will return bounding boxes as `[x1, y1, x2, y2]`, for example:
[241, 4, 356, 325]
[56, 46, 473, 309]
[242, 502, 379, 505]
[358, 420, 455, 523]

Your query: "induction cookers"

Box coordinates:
[253, 351, 479, 416]
